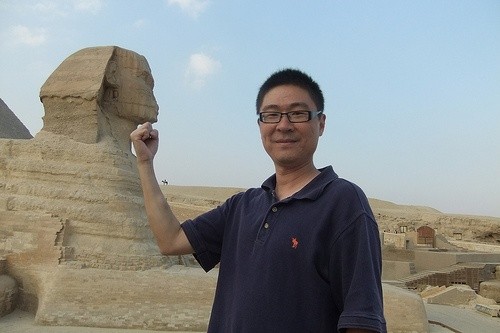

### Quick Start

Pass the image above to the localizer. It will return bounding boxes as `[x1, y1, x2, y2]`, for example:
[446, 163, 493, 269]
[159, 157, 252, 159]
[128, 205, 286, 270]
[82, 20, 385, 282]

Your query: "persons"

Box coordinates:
[127, 68, 391, 333]
[36, 46, 161, 154]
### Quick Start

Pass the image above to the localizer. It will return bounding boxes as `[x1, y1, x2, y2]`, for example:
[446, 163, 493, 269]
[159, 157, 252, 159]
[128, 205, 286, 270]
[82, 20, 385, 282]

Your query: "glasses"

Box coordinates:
[259, 109, 322, 123]
